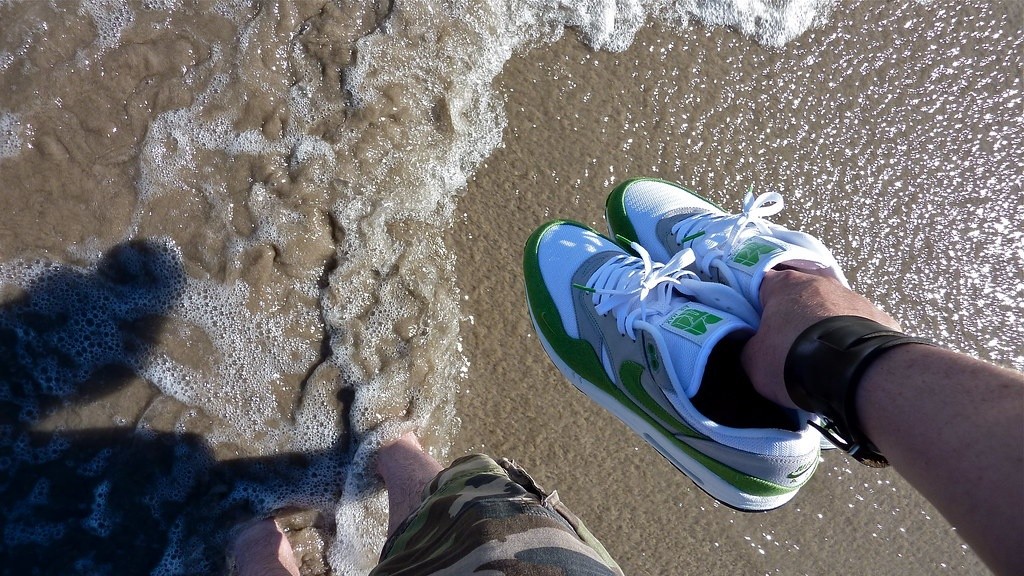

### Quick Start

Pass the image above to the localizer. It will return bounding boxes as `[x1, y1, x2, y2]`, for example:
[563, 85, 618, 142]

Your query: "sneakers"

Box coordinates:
[523, 219, 821, 513]
[605, 176, 850, 451]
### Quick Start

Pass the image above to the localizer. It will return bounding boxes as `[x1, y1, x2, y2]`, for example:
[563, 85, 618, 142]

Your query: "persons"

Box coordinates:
[234, 269, 1024, 576]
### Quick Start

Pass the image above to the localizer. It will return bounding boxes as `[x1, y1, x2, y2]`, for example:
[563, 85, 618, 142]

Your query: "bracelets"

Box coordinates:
[784, 316, 943, 469]
